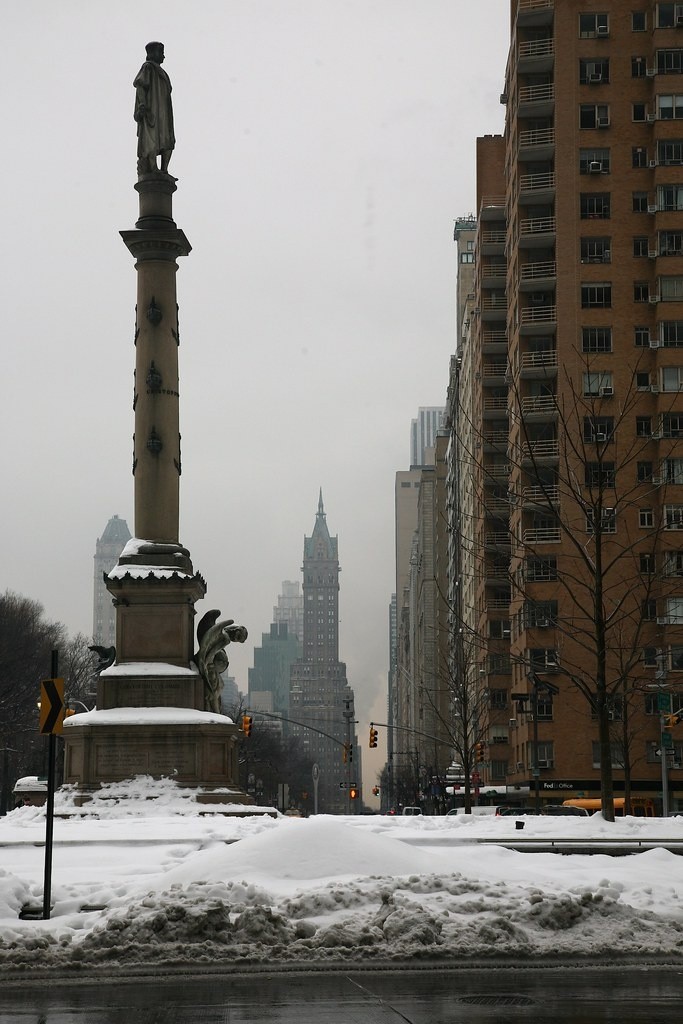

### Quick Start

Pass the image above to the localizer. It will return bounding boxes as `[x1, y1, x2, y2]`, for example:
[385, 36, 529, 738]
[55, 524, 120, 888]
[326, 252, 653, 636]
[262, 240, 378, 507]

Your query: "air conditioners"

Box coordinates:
[536, 619, 550, 627]
[503, 630, 510, 640]
[509, 719, 516, 728]
[590, 162, 601, 175]
[593, 433, 606, 444]
[505, 374, 512, 383]
[676, 15, 683, 27]
[604, 507, 616, 517]
[646, 69, 663, 488]
[654, 616, 667, 627]
[545, 662, 559, 672]
[598, 26, 609, 38]
[538, 760, 551, 769]
[608, 711, 614, 721]
[500, 94, 508, 104]
[504, 465, 513, 473]
[598, 117, 610, 129]
[599, 387, 614, 400]
[590, 73, 602, 85]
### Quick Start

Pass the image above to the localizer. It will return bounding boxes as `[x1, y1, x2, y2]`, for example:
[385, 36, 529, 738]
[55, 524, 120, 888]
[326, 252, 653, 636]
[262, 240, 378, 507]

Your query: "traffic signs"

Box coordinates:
[340, 781, 356, 789]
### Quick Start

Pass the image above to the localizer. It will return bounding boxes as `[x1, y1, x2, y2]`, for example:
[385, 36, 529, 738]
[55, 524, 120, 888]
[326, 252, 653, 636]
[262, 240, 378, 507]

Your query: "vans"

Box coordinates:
[402, 807, 423, 817]
[445, 805, 503, 818]
[563, 796, 656, 820]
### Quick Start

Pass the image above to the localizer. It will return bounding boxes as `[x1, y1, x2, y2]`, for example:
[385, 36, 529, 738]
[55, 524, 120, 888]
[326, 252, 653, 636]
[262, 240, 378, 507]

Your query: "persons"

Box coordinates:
[134, 40, 179, 182]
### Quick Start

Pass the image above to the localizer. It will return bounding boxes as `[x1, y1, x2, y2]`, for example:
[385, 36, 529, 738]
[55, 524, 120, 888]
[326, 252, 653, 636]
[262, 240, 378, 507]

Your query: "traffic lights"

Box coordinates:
[64, 709, 78, 717]
[350, 790, 359, 798]
[373, 788, 380, 797]
[370, 728, 378, 748]
[669, 715, 680, 727]
[344, 744, 353, 764]
[475, 743, 484, 763]
[241, 714, 252, 738]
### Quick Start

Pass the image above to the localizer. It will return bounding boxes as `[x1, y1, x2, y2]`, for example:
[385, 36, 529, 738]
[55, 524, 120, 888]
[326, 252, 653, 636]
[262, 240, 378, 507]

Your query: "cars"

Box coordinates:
[499, 806, 591, 817]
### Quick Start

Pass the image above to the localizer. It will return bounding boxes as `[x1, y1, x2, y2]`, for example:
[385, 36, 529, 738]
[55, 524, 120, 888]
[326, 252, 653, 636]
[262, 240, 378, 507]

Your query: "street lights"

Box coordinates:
[524, 671, 561, 818]
